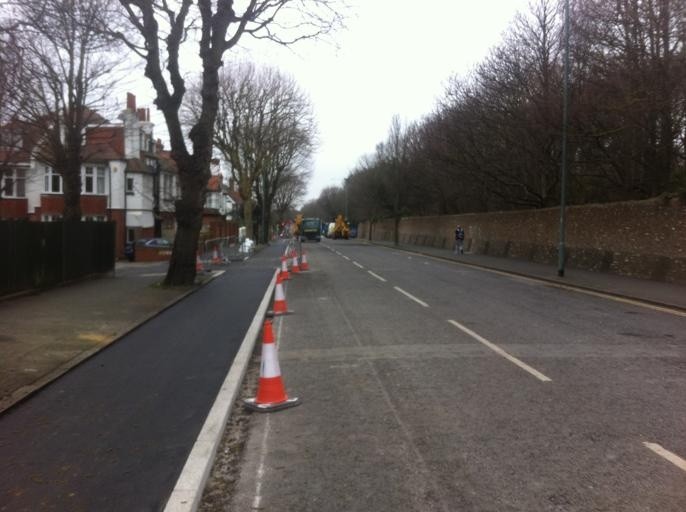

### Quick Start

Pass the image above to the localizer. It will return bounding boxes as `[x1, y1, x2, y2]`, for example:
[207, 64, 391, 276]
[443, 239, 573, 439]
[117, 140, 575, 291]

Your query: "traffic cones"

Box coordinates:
[196, 243, 221, 273]
[241, 247, 310, 412]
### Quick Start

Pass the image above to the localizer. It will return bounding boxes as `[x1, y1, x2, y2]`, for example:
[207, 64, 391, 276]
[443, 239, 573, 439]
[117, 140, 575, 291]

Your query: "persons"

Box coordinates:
[454, 224, 464, 256]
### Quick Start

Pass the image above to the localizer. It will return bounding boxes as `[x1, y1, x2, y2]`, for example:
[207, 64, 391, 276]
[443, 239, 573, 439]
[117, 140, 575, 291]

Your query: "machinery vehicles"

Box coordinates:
[333, 214, 350, 239]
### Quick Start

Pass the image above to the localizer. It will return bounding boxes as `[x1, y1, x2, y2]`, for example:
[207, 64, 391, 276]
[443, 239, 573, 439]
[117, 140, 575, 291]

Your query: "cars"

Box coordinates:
[125, 238, 172, 261]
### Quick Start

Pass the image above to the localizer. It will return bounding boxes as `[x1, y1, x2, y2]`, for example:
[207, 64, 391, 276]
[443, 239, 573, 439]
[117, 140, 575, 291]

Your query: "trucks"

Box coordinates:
[300, 218, 320, 242]
